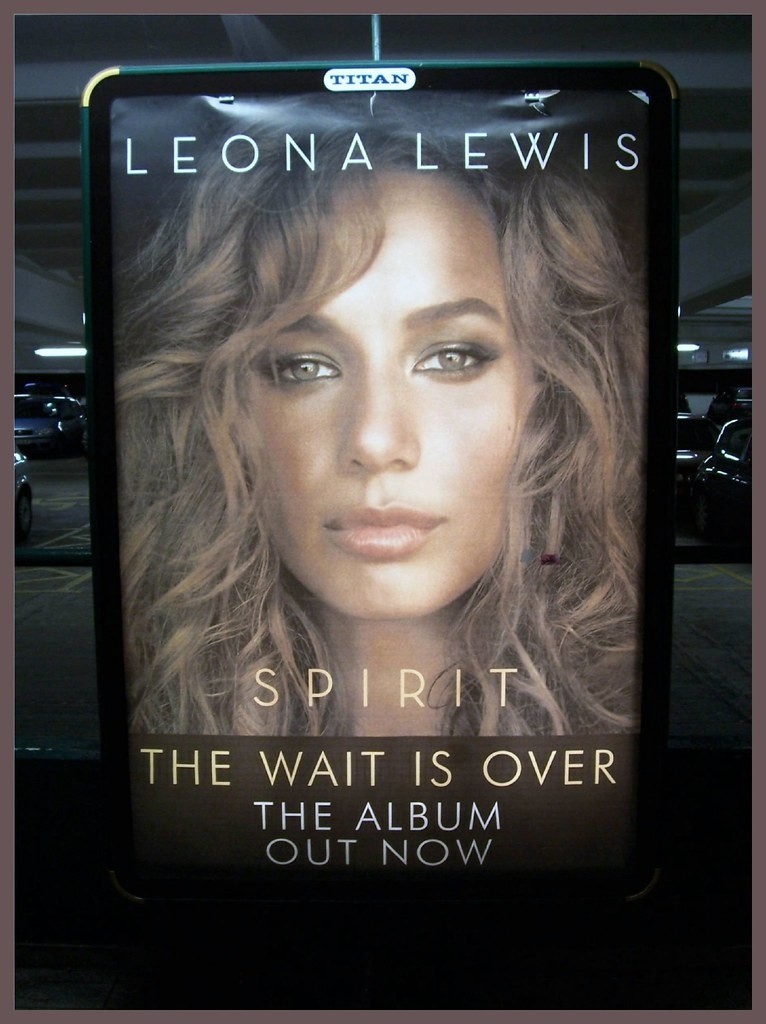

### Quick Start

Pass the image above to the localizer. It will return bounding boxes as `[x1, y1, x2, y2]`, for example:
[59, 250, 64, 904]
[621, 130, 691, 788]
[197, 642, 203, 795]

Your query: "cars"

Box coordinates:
[675, 412, 723, 506]
[14, 446, 34, 539]
[15, 395, 87, 459]
[688, 417, 752, 553]
[705, 385, 752, 429]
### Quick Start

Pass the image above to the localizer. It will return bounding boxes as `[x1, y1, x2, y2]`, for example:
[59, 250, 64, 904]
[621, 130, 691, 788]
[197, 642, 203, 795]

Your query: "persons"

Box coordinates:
[116, 92, 643, 737]
